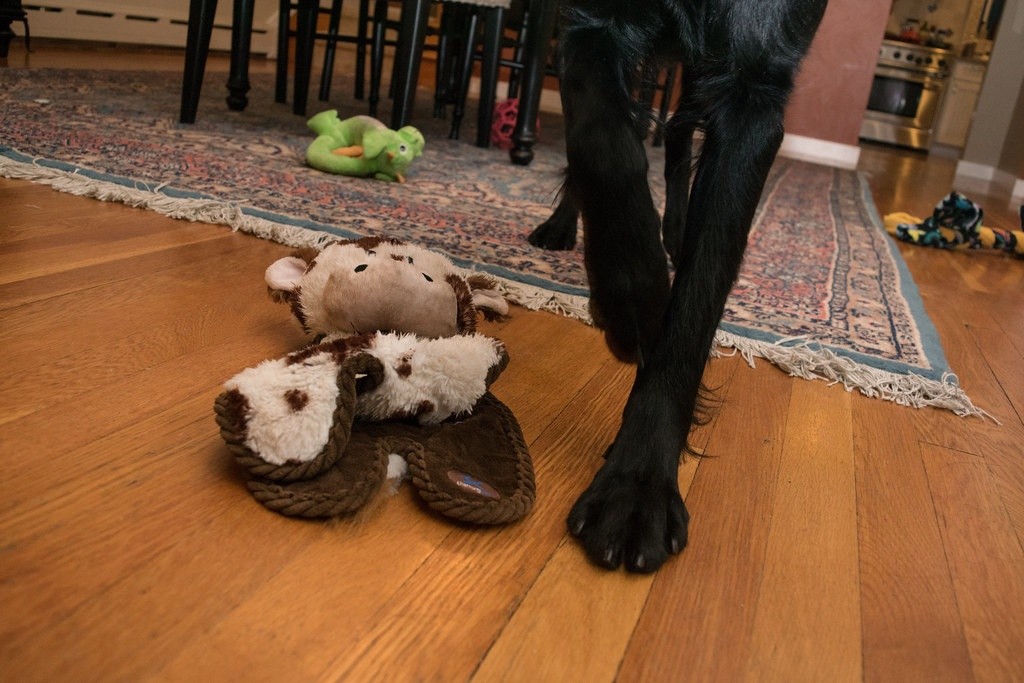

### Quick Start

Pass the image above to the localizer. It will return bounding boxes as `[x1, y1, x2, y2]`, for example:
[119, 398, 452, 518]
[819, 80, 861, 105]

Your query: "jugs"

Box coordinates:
[927, 25, 949, 45]
[902, 18, 927, 38]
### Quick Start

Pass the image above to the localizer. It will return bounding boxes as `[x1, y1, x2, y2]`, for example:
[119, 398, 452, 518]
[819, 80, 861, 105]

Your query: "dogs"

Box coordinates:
[525, 1, 827, 578]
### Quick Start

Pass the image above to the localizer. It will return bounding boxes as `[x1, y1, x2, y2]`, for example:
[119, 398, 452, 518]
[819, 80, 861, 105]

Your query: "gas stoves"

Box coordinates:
[875, 32, 955, 74]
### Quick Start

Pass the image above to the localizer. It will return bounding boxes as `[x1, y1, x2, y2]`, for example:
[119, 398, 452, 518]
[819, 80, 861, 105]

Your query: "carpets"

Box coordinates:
[0, 61, 1014, 428]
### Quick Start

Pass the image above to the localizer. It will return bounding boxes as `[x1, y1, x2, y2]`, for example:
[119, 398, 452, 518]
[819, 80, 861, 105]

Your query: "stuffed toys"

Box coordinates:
[305, 108, 425, 184]
[882, 189, 1024, 260]
[212, 233, 536, 527]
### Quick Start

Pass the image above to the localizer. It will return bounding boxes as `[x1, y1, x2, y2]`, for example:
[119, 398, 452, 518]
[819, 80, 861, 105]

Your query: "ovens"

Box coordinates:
[855, 59, 946, 152]
